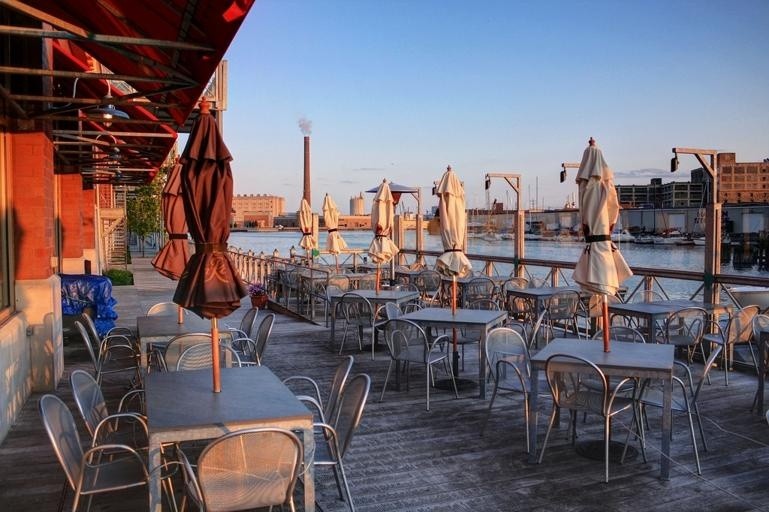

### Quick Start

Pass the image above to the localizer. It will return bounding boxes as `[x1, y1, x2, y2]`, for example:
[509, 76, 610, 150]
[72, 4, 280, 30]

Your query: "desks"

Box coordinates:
[146, 364, 315, 511]
[530, 339, 675, 480]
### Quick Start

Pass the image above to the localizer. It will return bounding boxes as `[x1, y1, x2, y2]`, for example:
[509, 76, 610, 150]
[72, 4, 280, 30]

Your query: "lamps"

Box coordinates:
[26, 75, 130, 181]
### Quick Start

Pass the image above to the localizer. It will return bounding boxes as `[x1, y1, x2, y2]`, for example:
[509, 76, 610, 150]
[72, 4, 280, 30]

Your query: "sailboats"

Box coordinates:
[462, 172, 757, 248]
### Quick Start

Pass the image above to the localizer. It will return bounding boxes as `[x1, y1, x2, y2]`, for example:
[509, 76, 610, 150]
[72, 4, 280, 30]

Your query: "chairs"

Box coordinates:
[481, 327, 578, 453]
[281, 355, 356, 498]
[40, 396, 178, 508]
[76, 301, 277, 432]
[176, 427, 301, 511]
[620, 345, 723, 476]
[269, 371, 371, 511]
[538, 354, 649, 482]
[278, 255, 768, 416]
[70, 369, 178, 508]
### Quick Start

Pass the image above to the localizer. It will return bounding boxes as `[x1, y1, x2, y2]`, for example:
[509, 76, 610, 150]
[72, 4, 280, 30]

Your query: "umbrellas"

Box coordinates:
[299, 196, 316, 266]
[323, 192, 347, 270]
[152, 154, 192, 325]
[362, 179, 399, 351]
[173, 95, 248, 392]
[572, 137, 640, 461]
[431, 165, 478, 390]
